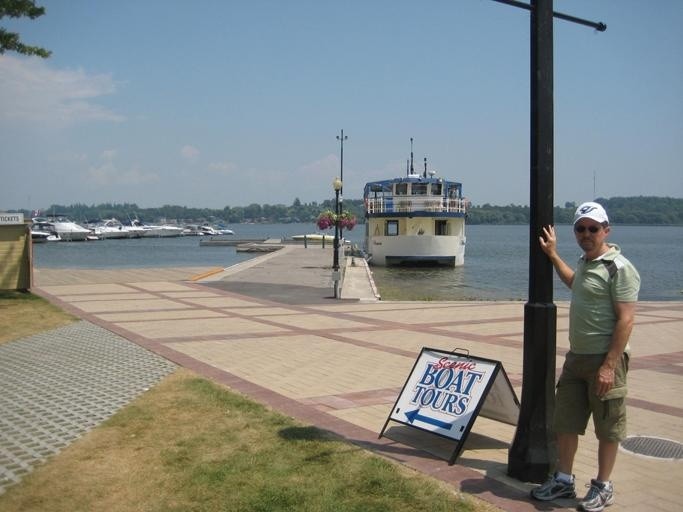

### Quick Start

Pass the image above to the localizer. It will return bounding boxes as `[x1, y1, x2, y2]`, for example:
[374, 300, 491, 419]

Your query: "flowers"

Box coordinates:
[314, 210, 356, 232]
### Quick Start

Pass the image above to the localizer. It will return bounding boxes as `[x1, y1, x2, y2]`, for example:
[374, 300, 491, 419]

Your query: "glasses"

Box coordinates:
[576, 224, 605, 234]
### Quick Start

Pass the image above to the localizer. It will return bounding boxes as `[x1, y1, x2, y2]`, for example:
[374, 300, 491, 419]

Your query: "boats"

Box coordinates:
[363, 136, 465, 269]
[27, 215, 236, 242]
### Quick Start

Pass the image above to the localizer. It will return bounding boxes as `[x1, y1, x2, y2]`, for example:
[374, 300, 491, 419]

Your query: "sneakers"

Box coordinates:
[529, 471, 577, 500]
[578, 479, 614, 511]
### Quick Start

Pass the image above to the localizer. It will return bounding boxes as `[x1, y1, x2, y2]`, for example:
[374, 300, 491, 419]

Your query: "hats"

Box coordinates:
[572, 202, 610, 224]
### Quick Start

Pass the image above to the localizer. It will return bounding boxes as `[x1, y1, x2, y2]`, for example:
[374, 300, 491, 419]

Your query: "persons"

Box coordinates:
[530, 202, 642, 511]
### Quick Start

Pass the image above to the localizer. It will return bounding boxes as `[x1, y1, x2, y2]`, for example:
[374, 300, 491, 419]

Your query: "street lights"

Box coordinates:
[332, 174, 341, 268]
[333, 127, 347, 240]
